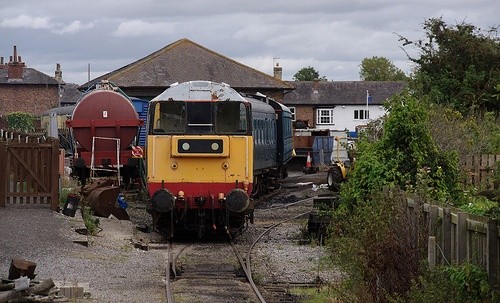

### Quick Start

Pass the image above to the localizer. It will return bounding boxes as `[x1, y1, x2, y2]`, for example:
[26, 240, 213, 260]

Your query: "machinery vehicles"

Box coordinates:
[327, 133, 361, 191]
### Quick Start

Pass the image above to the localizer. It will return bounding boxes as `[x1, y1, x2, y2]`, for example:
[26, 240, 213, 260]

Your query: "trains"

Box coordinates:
[67, 78, 148, 188]
[144, 80, 295, 240]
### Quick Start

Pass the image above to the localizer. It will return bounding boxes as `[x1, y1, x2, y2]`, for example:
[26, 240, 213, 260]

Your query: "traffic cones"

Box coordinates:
[306, 151, 312, 168]
